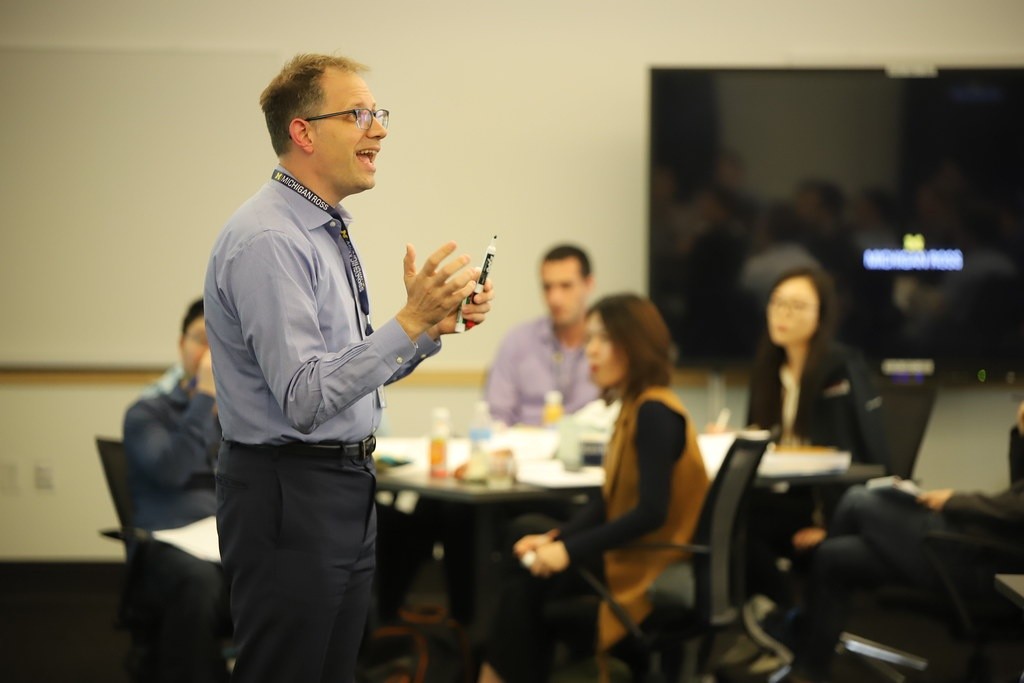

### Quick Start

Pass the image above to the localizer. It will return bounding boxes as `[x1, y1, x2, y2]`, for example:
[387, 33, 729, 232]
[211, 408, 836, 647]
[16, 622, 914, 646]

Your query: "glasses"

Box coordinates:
[288, 107, 389, 140]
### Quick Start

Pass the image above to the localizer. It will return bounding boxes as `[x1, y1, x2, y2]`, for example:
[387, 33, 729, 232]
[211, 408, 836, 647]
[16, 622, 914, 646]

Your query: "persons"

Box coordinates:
[477, 294, 708, 682]
[203, 53, 491, 683]
[790, 400, 1022, 683]
[481, 245, 605, 428]
[720, 268, 889, 673]
[121, 295, 225, 683]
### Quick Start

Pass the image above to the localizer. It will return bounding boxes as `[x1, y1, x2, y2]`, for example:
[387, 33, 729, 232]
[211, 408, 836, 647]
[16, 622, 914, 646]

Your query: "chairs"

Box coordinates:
[93, 436, 237, 683]
[868, 357, 939, 486]
[566, 431, 794, 683]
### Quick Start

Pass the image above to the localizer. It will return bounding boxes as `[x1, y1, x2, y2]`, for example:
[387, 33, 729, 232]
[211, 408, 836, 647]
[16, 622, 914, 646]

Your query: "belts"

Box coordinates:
[279, 435, 377, 459]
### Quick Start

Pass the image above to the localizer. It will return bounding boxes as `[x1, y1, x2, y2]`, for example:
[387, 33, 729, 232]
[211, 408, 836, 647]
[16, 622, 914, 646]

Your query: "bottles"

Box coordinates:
[427, 407, 448, 474]
[544, 390, 561, 431]
[470, 403, 493, 456]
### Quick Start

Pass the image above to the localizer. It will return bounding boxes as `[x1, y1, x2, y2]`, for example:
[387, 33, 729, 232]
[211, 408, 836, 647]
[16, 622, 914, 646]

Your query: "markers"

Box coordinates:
[467, 235, 498, 294]
[454, 268, 481, 332]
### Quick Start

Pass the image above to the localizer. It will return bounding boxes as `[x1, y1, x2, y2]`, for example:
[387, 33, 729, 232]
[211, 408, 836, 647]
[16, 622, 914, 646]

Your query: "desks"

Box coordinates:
[364, 430, 889, 630]
